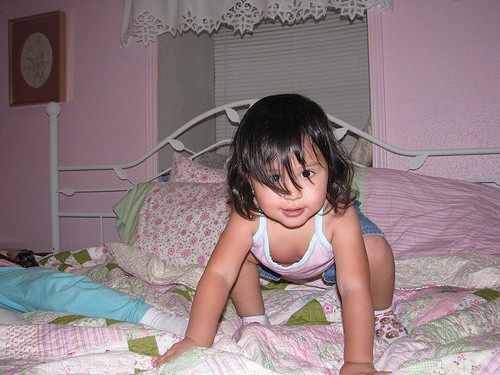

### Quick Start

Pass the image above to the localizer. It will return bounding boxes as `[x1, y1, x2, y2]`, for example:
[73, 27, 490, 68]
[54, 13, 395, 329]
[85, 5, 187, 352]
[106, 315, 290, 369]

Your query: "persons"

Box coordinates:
[152, 94, 395, 375]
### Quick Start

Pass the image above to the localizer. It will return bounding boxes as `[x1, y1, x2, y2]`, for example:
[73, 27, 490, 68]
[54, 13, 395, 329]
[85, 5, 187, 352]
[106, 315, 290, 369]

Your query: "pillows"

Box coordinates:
[347, 165, 500, 259]
[127, 151, 231, 267]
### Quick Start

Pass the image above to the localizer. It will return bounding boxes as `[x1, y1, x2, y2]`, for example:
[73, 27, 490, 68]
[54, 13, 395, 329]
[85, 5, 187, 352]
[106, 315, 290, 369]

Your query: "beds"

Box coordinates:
[0, 96, 500, 375]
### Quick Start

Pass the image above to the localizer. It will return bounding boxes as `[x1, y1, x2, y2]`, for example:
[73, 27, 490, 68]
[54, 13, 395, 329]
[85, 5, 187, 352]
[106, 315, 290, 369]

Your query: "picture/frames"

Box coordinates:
[6, 10, 68, 107]
